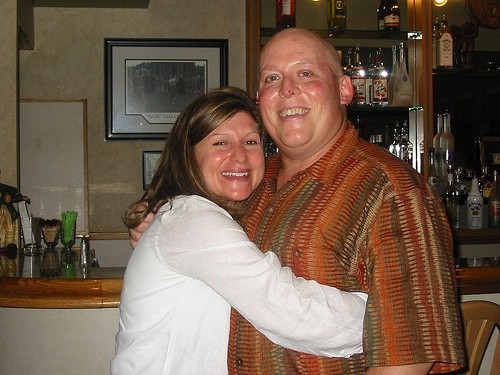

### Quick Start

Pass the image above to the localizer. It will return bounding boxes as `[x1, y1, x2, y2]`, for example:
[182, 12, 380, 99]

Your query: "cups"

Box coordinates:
[21, 217, 41, 256]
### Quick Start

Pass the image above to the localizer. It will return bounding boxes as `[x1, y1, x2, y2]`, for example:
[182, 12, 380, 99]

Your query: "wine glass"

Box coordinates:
[42, 226, 59, 251]
[60, 220, 76, 253]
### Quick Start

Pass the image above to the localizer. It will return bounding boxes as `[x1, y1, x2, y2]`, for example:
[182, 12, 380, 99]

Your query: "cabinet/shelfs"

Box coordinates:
[247, 1, 499, 242]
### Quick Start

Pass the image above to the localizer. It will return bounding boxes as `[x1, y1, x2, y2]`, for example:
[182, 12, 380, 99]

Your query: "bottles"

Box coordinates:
[368, 128, 413, 166]
[80, 234, 90, 254]
[443, 166, 500, 229]
[433, 112, 454, 178]
[432, 13, 455, 70]
[275, 0, 400, 32]
[343, 42, 413, 106]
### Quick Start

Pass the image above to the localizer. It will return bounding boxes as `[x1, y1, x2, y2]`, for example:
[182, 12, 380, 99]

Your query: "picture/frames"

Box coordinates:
[142, 150, 163, 191]
[103, 37, 229, 138]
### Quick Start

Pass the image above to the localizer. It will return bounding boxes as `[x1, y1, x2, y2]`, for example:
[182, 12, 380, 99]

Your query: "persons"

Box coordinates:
[111, 86, 371, 375]
[127, 29, 468, 375]
